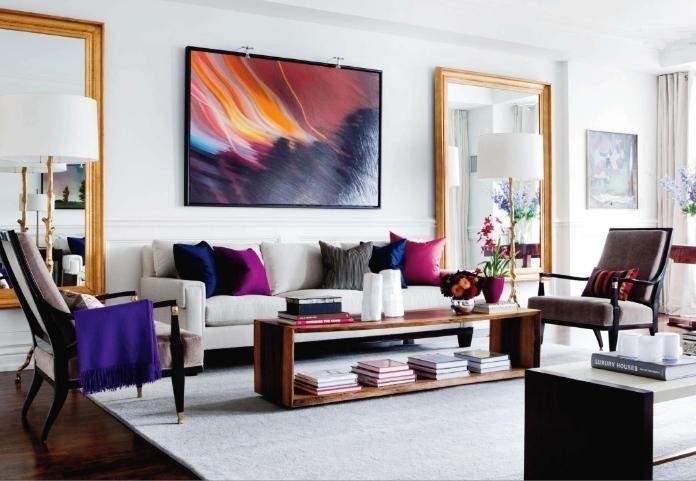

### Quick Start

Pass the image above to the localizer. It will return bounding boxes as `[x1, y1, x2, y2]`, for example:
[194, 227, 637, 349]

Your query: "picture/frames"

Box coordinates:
[39, 163, 86, 209]
[585, 135, 638, 209]
[184, 45, 383, 207]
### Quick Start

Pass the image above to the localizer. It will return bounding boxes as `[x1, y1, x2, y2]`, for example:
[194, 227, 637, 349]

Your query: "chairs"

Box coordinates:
[528, 226, 674, 349]
[0, 231, 201, 442]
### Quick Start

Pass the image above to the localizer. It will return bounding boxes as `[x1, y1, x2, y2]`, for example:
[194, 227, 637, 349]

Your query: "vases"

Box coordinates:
[480, 278, 505, 303]
[450, 299, 476, 315]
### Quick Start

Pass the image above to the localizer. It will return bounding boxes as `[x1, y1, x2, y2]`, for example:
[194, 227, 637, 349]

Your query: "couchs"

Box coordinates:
[142, 246, 476, 376]
[55, 234, 86, 283]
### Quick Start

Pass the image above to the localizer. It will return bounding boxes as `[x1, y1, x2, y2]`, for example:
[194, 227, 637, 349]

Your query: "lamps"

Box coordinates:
[237, 46, 254, 60]
[477, 133, 542, 306]
[0, 97, 68, 233]
[19, 191, 50, 249]
[21, 93, 98, 278]
[325, 57, 344, 69]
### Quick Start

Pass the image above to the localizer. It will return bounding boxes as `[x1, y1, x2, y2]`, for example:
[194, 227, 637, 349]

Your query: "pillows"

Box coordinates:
[173, 241, 231, 297]
[319, 240, 374, 291]
[389, 230, 447, 286]
[65, 235, 86, 263]
[581, 267, 639, 301]
[360, 238, 408, 289]
[213, 243, 270, 296]
[59, 290, 105, 311]
[153, 240, 178, 277]
[260, 243, 325, 296]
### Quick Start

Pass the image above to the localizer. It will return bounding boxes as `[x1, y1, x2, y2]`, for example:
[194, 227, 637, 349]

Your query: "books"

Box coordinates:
[454, 349, 511, 374]
[406, 353, 471, 380]
[294, 369, 361, 396]
[276, 311, 354, 326]
[349, 359, 416, 388]
[591, 352, 696, 381]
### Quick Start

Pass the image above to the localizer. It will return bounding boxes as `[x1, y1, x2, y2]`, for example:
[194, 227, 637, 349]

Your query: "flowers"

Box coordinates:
[491, 178, 540, 229]
[470, 214, 520, 277]
[441, 271, 482, 300]
[659, 168, 696, 218]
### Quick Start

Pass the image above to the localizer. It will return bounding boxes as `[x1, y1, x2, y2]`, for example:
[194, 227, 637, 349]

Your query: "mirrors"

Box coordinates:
[4, 7, 106, 310]
[435, 66, 553, 284]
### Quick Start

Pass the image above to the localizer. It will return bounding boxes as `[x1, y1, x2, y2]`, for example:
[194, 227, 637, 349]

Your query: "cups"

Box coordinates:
[640, 335, 664, 362]
[655, 332, 681, 361]
[619, 332, 639, 357]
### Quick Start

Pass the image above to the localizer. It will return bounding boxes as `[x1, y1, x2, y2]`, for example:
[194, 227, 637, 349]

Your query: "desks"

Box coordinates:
[526, 339, 696, 481]
